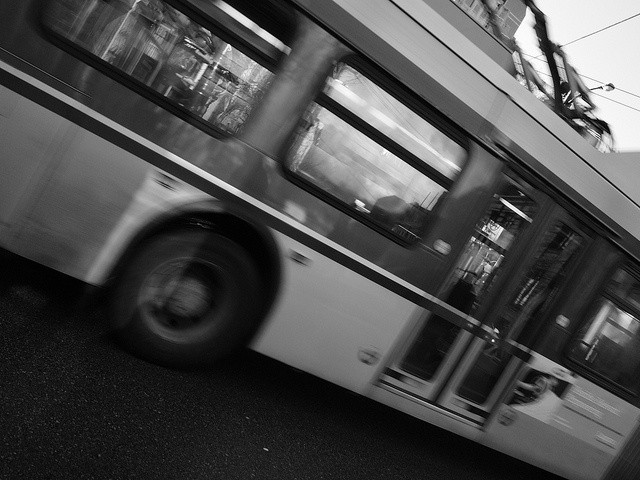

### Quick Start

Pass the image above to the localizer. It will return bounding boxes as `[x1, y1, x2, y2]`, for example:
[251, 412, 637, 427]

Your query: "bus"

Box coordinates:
[0, 0, 640, 480]
